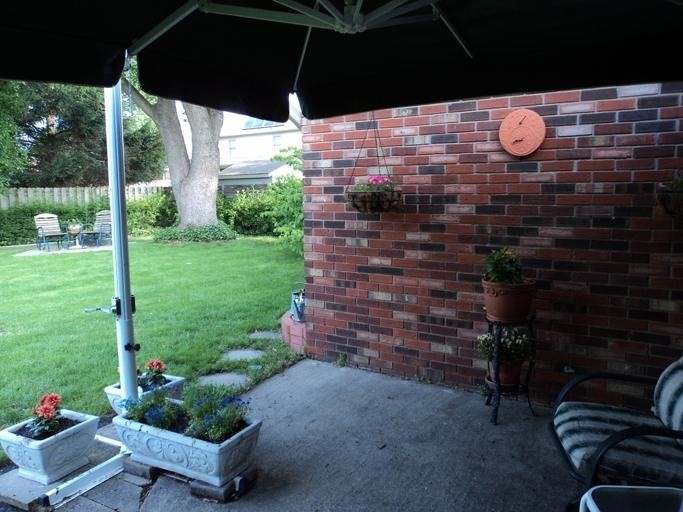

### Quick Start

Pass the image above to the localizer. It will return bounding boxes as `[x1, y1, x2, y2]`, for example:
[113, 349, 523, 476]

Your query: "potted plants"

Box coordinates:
[113, 382, 263, 488]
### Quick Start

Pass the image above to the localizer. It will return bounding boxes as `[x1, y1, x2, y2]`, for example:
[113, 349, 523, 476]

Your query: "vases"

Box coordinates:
[484, 355, 523, 390]
[103, 374, 185, 415]
[345, 190, 402, 214]
[0, 409, 101, 486]
[482, 279, 538, 319]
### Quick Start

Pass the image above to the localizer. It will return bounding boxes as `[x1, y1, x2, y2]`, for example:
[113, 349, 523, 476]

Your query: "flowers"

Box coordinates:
[485, 247, 526, 282]
[28, 387, 63, 437]
[476, 327, 530, 363]
[350, 171, 395, 192]
[138, 358, 167, 390]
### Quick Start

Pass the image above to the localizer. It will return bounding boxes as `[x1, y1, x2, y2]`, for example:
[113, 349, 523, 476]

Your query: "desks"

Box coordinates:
[68, 230, 87, 249]
[579, 483, 683, 511]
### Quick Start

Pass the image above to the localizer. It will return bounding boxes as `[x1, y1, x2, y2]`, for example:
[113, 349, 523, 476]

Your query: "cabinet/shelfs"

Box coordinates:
[481, 313, 538, 425]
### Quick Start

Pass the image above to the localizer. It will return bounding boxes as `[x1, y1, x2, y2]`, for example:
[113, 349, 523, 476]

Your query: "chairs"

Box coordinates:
[547, 354, 683, 495]
[80, 210, 112, 249]
[32, 213, 68, 250]
